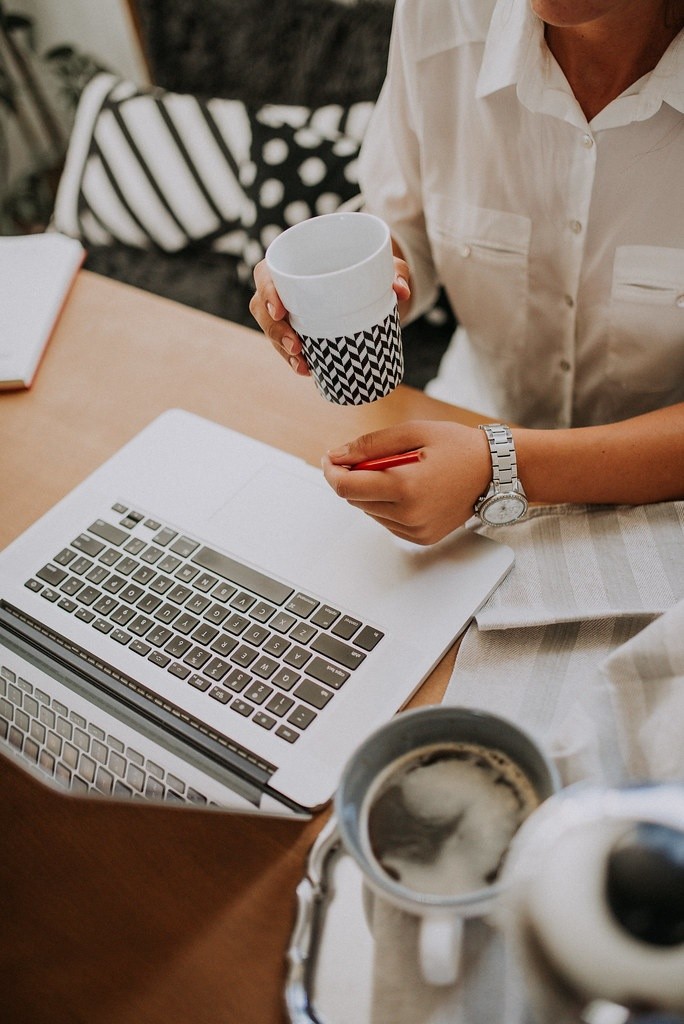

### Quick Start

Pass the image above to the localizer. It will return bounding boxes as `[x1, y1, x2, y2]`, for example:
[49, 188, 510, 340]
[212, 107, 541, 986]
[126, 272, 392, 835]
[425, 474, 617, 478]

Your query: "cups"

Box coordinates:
[266, 211, 405, 407]
[334, 703, 558, 987]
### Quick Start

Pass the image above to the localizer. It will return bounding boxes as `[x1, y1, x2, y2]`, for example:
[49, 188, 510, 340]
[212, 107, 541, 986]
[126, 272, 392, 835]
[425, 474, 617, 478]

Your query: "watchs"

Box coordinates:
[473, 423, 530, 528]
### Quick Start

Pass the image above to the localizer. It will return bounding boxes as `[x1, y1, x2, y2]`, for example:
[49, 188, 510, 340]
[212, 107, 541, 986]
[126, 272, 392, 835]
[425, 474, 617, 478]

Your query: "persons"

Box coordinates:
[249, 0, 683, 545]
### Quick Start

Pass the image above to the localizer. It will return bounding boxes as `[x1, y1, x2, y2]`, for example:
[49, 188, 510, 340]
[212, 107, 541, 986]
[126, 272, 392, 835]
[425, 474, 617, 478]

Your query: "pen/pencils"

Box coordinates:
[351, 449, 428, 475]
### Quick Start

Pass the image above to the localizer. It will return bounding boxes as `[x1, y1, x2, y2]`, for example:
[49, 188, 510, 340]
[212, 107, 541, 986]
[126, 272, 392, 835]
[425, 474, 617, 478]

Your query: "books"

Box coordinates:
[0, 232, 88, 391]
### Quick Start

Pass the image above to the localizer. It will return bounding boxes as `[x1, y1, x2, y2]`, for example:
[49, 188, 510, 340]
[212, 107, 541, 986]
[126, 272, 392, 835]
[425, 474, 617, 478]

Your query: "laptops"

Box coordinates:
[0, 408, 518, 822]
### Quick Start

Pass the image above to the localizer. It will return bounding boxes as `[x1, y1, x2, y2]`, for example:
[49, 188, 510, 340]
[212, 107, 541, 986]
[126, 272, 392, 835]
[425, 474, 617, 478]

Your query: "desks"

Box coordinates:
[0, 269, 529, 1024]
